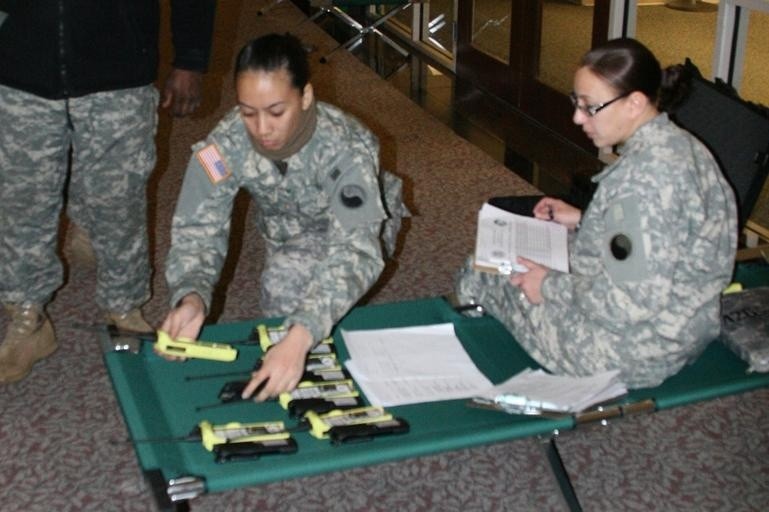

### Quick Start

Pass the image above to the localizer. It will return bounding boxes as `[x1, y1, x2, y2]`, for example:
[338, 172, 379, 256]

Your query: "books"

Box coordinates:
[478, 368, 629, 416]
[473, 202, 572, 277]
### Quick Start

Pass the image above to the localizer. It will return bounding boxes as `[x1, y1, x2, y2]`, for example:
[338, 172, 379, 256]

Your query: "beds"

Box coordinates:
[95, 257, 769, 512]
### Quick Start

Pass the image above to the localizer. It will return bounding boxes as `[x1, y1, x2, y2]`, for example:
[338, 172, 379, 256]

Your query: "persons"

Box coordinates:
[152, 29, 417, 405]
[453, 36, 742, 390]
[0, 0, 215, 384]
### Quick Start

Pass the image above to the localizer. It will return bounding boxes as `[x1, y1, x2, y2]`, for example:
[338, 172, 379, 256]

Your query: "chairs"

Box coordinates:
[487, 58, 767, 233]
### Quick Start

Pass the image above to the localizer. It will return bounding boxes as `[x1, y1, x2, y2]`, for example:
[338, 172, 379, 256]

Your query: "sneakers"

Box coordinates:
[105, 307, 157, 342]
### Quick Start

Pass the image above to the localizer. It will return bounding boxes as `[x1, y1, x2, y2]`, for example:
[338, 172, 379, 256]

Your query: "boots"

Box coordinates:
[0, 299, 59, 384]
